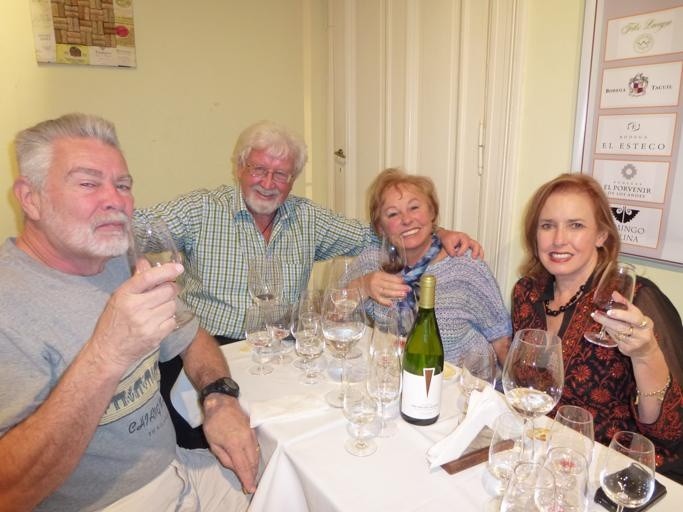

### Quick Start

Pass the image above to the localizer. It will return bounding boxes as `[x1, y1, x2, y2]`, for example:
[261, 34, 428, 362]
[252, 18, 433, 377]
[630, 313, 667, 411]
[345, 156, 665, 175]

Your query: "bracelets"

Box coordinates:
[634, 374, 671, 398]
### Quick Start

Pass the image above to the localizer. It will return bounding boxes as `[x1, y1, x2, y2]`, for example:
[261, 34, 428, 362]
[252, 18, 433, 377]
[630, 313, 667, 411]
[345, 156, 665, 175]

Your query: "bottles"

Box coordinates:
[397, 274, 444, 426]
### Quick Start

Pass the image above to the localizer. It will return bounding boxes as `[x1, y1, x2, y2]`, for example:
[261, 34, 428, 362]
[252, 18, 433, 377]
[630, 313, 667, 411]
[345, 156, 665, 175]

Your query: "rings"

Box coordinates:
[625, 327, 634, 335]
[614, 333, 624, 340]
[638, 320, 647, 326]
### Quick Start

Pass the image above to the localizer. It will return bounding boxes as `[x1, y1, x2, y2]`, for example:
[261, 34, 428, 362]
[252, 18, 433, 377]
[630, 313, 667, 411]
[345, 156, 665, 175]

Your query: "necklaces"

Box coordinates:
[261, 213, 276, 234]
[541, 268, 586, 317]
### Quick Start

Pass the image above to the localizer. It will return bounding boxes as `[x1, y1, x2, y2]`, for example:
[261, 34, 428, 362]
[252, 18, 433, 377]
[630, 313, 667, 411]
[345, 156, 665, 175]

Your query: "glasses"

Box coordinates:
[246, 165, 295, 186]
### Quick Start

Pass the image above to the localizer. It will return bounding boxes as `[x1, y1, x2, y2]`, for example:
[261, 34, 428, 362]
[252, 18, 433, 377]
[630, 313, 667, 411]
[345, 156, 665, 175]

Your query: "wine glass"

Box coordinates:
[582, 261, 636, 348]
[124, 217, 191, 335]
[457, 322, 657, 512]
[241, 251, 403, 456]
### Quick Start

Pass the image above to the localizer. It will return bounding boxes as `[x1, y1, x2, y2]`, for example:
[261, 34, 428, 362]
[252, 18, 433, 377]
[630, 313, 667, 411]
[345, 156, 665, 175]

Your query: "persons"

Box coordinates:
[335, 168, 511, 392]
[508, 172, 681, 482]
[124, 120, 487, 348]
[0, 115, 261, 511]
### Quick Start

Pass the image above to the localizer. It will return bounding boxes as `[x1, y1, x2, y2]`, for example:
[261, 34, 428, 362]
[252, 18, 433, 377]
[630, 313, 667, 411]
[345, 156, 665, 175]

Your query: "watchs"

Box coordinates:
[196, 374, 239, 398]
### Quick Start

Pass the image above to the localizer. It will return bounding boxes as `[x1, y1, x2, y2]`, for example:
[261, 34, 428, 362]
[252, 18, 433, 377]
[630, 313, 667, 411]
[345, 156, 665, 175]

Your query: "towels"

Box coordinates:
[423, 379, 507, 468]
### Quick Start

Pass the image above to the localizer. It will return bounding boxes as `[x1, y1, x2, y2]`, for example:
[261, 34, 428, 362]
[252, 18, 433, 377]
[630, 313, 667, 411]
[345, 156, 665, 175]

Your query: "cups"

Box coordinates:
[379, 234, 406, 274]
